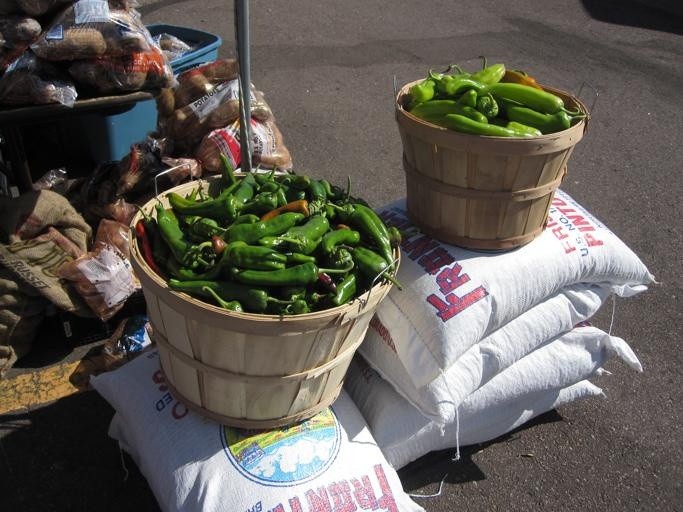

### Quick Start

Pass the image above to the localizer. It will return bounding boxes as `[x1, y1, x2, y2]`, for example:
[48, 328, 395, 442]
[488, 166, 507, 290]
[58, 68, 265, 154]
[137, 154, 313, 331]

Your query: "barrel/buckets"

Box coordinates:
[393, 70, 598, 249]
[126, 162, 401, 431]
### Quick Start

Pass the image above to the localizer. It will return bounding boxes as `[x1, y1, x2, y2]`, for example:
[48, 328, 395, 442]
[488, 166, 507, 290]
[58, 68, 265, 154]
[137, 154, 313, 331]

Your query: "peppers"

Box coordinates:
[409, 55, 587, 138]
[135, 152, 403, 315]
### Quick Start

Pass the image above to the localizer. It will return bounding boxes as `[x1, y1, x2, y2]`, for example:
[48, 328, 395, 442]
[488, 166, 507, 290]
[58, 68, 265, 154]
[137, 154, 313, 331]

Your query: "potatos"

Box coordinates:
[0, 0, 291, 172]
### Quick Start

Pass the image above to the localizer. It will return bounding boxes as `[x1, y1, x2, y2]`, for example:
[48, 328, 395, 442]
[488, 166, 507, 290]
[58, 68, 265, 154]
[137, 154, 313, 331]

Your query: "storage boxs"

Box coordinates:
[21, 23, 224, 175]
